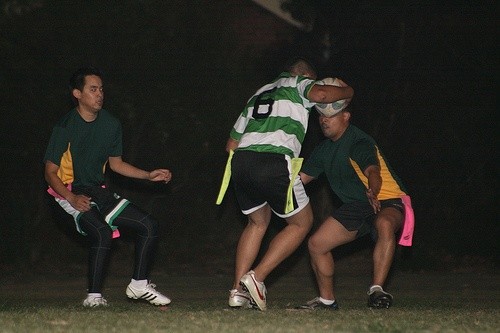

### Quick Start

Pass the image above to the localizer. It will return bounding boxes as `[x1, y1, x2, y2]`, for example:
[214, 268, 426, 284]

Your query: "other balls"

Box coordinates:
[312, 77, 352, 118]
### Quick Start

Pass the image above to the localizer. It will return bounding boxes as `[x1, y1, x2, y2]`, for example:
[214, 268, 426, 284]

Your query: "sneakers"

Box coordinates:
[82, 295, 109, 308]
[125, 279, 171, 305]
[286, 296, 338, 311]
[366, 285, 395, 309]
[239, 270, 268, 311]
[228, 289, 257, 309]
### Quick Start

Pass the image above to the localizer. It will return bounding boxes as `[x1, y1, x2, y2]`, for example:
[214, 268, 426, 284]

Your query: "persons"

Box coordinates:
[299, 108, 409, 311]
[45, 68, 172, 307]
[225, 55, 354, 312]
